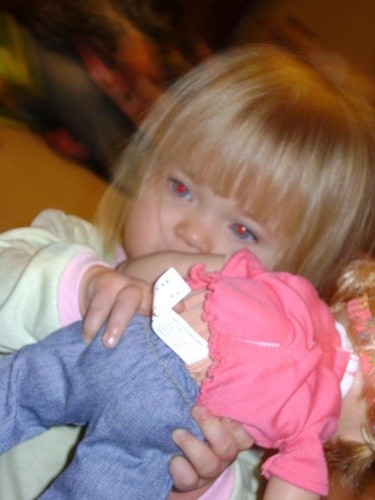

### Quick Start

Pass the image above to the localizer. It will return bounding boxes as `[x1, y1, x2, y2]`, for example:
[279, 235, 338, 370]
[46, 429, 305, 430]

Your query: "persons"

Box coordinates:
[0, 43, 374, 500]
[0, 244, 374, 500]
